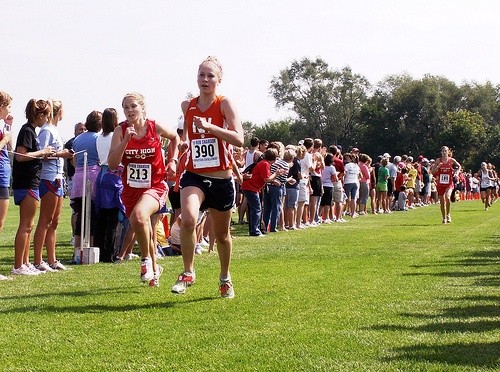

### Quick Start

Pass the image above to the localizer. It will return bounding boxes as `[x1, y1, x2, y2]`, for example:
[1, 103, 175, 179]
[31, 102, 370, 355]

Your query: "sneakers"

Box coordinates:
[11, 263, 46, 276]
[67, 195, 480, 266]
[0, 273, 8, 280]
[484, 195, 500, 211]
[218, 271, 235, 298]
[35, 262, 58, 273]
[140, 256, 164, 287]
[170, 271, 196, 294]
[48, 261, 68, 270]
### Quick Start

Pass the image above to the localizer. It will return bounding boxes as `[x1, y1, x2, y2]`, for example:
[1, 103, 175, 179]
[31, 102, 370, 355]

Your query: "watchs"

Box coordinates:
[169, 156, 179, 165]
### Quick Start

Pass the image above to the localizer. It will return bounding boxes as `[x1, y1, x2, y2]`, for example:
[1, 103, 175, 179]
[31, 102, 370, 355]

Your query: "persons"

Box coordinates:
[229, 135, 440, 239]
[151, 209, 183, 257]
[12, 97, 57, 276]
[107, 92, 180, 288]
[0, 90, 15, 283]
[70, 110, 108, 265]
[64, 122, 99, 244]
[431, 146, 461, 224]
[34, 99, 77, 275]
[145, 127, 171, 253]
[94, 105, 132, 262]
[115, 215, 141, 263]
[446, 147, 500, 213]
[166, 121, 191, 236]
[169, 55, 245, 303]
[168, 201, 211, 259]
